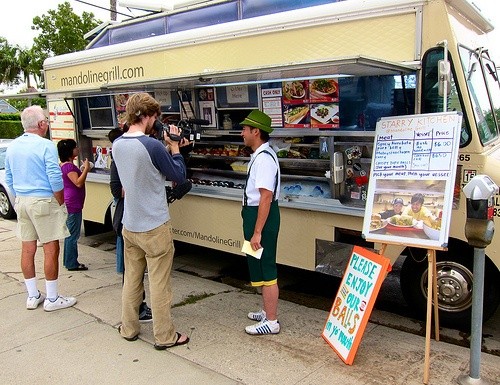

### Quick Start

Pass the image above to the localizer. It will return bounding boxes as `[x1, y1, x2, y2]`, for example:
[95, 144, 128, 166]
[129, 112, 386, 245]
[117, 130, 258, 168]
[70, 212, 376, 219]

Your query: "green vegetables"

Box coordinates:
[317, 107, 328, 112]
[315, 79, 334, 92]
[286, 81, 304, 117]
[276, 150, 288, 158]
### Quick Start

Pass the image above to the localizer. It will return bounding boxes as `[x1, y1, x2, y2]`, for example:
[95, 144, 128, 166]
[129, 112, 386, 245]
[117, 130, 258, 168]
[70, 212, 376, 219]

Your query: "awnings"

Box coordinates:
[0, 54, 420, 102]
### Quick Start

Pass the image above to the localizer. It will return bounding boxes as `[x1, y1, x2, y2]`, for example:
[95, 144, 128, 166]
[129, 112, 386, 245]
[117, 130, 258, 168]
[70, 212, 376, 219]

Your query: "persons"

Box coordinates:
[4, 105, 77, 311]
[241, 109, 281, 335]
[374, 193, 437, 230]
[58, 138, 91, 271]
[103, 93, 192, 350]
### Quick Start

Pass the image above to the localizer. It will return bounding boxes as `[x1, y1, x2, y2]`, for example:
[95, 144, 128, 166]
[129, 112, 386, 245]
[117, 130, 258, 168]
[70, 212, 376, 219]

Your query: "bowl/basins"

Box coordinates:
[423, 223, 440, 241]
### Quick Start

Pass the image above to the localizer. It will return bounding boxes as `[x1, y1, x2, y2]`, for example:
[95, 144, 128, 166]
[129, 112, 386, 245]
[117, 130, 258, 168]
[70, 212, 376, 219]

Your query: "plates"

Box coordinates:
[310, 103, 338, 123]
[283, 107, 309, 124]
[311, 83, 336, 95]
[387, 217, 417, 227]
[369, 219, 388, 232]
[285, 82, 305, 98]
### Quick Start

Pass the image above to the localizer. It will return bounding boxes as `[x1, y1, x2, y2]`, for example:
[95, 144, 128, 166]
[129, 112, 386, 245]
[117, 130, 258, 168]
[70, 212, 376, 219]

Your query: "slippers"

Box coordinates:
[119, 325, 137, 340]
[155, 331, 189, 350]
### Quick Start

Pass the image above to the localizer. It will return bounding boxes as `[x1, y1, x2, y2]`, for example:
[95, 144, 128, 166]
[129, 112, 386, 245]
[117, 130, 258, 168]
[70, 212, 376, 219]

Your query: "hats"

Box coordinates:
[394, 198, 404, 205]
[240, 110, 273, 133]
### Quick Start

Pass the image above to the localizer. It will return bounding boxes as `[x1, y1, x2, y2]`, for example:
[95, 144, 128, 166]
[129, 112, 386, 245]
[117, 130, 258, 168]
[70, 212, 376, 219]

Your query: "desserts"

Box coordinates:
[190, 146, 251, 156]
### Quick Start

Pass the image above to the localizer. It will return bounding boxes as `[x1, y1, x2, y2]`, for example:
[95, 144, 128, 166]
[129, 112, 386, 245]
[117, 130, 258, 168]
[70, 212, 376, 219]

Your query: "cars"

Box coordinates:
[0, 139, 21, 219]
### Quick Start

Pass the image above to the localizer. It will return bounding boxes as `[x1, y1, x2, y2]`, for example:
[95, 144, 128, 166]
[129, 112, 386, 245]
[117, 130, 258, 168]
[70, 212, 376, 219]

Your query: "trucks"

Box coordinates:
[37, 0, 500, 329]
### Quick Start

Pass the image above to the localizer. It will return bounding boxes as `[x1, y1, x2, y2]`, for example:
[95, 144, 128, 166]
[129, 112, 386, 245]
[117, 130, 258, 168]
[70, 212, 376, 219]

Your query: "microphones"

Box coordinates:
[186, 119, 209, 126]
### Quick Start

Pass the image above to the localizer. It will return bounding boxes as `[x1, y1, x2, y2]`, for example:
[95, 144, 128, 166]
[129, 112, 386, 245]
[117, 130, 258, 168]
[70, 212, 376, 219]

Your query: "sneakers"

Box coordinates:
[244, 318, 281, 335]
[44, 296, 76, 311]
[26, 290, 46, 310]
[138, 301, 153, 322]
[247, 309, 267, 321]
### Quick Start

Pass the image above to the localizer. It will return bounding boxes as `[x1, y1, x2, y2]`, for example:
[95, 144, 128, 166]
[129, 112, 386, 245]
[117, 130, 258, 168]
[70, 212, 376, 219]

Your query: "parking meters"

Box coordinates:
[458, 174, 499, 384]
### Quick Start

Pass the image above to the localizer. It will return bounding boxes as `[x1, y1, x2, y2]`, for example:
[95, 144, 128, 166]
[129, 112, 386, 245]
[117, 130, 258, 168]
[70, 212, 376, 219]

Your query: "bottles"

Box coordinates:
[223, 115, 232, 129]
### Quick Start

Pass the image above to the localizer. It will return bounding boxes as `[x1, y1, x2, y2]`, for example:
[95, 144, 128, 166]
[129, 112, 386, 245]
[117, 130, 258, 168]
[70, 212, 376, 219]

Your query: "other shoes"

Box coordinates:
[68, 263, 88, 271]
